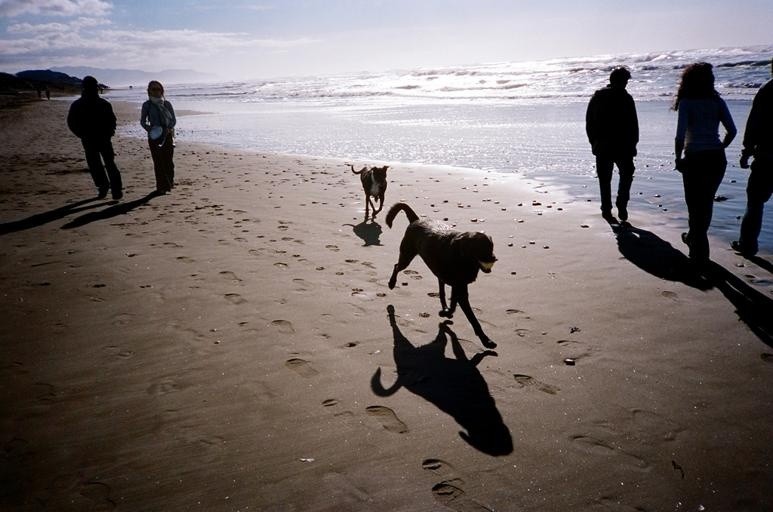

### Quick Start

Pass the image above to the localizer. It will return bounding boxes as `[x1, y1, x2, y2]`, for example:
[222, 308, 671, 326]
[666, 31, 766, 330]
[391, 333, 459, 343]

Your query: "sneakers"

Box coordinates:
[680, 230, 689, 241]
[616, 202, 627, 223]
[730, 239, 757, 255]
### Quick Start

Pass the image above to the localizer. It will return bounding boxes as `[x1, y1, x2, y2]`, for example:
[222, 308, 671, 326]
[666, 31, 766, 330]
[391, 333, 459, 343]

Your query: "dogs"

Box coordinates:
[351, 164, 390, 219]
[384, 201, 499, 348]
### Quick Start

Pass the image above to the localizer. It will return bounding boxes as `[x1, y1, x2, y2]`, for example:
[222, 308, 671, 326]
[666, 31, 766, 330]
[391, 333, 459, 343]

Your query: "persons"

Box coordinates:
[585, 66, 641, 225]
[66, 76, 124, 201]
[138, 81, 179, 190]
[671, 62, 738, 274]
[729, 56, 773, 258]
